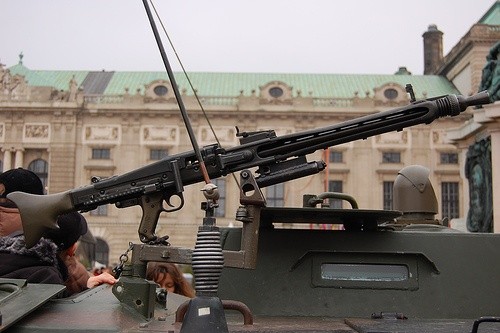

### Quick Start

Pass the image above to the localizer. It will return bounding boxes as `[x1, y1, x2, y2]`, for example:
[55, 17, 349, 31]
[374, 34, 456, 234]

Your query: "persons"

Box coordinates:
[62, 241, 122, 296]
[73, 228, 96, 271]
[0, 168, 47, 247]
[146, 259, 195, 299]
[100, 266, 106, 274]
[92, 268, 101, 275]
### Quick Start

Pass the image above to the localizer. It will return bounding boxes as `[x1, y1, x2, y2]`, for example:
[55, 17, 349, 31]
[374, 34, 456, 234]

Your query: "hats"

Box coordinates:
[0, 166, 43, 208]
[42, 208, 88, 252]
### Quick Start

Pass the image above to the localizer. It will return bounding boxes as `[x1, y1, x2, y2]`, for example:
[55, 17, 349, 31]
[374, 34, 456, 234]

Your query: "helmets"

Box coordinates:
[392, 164, 440, 215]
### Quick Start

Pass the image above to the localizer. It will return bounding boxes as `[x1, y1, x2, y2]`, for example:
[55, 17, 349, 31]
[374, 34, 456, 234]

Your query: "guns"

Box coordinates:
[6, 83, 491, 255]
